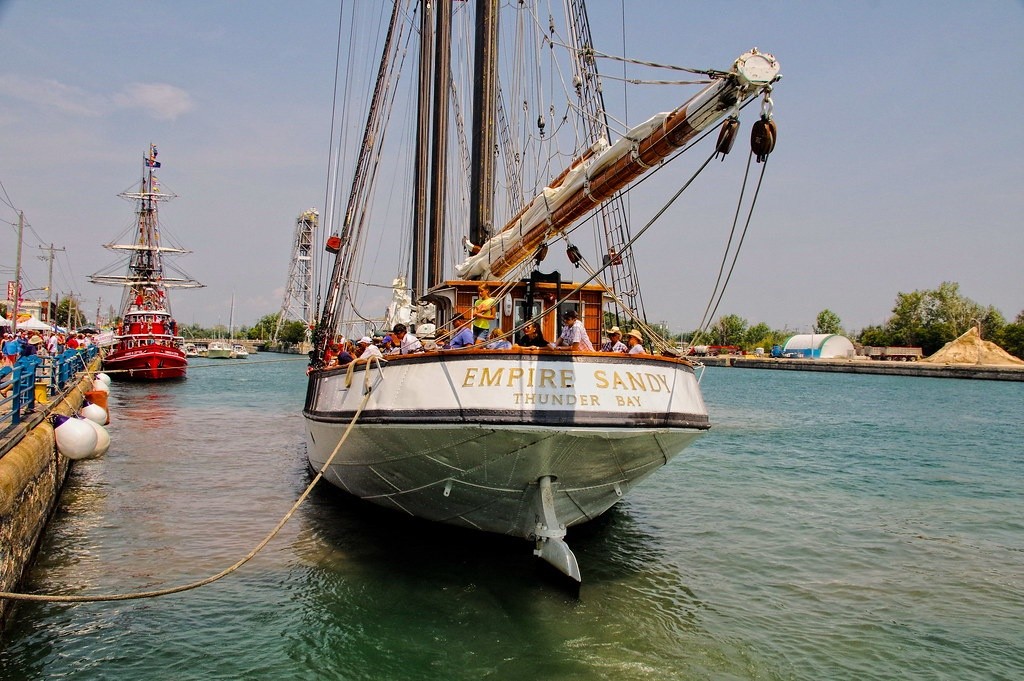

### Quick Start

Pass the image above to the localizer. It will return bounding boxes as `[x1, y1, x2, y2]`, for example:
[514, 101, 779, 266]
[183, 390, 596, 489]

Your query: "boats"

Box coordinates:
[182, 340, 259, 361]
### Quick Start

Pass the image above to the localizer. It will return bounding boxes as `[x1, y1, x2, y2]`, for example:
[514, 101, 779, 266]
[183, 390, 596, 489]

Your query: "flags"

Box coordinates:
[145, 158, 161, 168]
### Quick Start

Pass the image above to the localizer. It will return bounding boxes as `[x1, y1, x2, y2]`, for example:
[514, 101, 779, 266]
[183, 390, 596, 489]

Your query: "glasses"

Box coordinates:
[564, 317, 571, 320]
[526, 326, 530, 329]
[394, 332, 402, 335]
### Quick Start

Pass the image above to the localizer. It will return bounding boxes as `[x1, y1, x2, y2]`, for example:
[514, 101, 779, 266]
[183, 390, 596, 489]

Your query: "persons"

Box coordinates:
[306, 281, 651, 376]
[0, 329, 96, 413]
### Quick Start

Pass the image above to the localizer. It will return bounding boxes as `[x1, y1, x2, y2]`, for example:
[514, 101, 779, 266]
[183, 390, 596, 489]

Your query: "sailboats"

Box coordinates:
[88, 140, 207, 378]
[302, 0, 783, 581]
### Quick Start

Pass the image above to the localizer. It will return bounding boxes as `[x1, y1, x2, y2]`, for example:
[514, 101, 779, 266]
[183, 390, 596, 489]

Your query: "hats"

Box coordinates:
[27, 335, 43, 344]
[623, 329, 643, 344]
[356, 336, 373, 344]
[606, 326, 622, 341]
[562, 309, 582, 321]
[448, 313, 464, 320]
[382, 336, 391, 343]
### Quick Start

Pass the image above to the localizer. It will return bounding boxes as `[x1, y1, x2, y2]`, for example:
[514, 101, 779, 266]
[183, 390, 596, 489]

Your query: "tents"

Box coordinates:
[781, 334, 855, 359]
